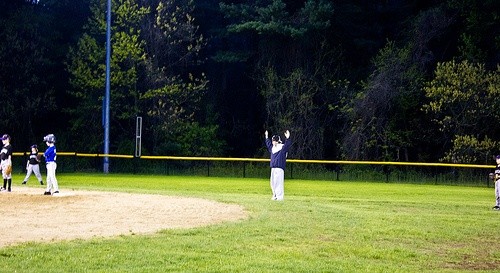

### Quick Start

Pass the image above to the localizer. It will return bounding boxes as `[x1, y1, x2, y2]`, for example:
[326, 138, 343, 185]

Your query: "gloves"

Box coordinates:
[265, 130, 268, 139]
[284, 130, 290, 139]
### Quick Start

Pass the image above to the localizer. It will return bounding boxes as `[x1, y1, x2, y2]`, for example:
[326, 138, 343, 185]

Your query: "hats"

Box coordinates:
[0, 134, 11, 140]
[495, 155, 500, 159]
[272, 136, 282, 143]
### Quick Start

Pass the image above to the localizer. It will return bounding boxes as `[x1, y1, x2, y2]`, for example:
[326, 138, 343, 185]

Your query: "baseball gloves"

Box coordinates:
[5, 166, 12, 175]
[37, 155, 42, 163]
[490, 173, 495, 181]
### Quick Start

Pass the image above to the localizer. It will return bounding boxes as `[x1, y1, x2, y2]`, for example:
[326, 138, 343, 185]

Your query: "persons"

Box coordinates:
[492, 154, 500, 211]
[43, 134, 59, 196]
[22, 145, 43, 185]
[265, 130, 292, 200]
[0, 134, 12, 192]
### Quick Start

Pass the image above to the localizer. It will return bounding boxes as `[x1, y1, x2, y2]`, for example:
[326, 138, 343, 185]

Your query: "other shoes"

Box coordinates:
[493, 205, 499, 209]
[22, 181, 26, 184]
[0, 187, 6, 192]
[40, 181, 44, 185]
[54, 190, 59, 193]
[44, 191, 51, 195]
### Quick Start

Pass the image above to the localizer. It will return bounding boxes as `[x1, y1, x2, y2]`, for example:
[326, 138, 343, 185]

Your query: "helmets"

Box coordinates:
[43, 134, 55, 142]
[30, 145, 38, 149]
